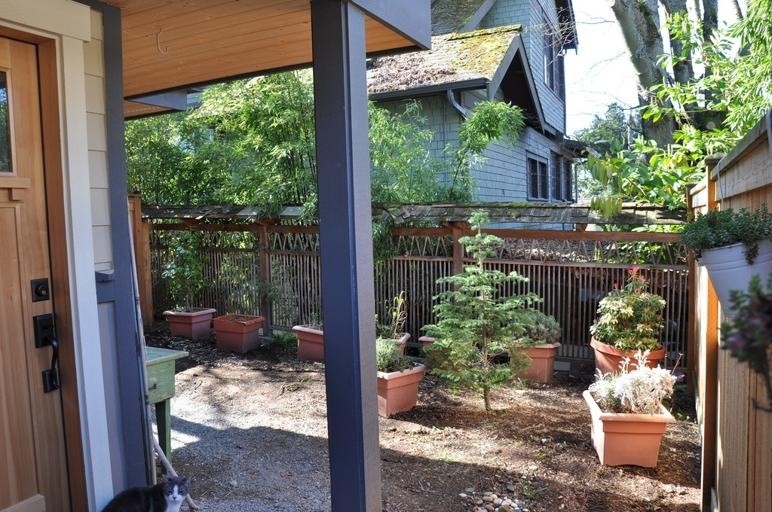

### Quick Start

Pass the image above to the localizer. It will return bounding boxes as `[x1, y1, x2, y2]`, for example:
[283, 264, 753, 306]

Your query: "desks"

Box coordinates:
[141, 344, 192, 479]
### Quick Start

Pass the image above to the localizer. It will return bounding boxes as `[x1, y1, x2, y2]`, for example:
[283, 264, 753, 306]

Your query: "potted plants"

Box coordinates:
[370, 286, 413, 359]
[287, 313, 325, 363]
[369, 336, 427, 421]
[680, 205, 772, 323]
[580, 347, 678, 471]
[156, 228, 218, 341]
[208, 252, 266, 357]
[417, 323, 469, 374]
[500, 306, 563, 387]
[587, 264, 669, 386]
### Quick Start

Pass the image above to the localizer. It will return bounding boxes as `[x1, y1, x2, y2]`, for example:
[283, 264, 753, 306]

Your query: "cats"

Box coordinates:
[101, 476, 192, 512]
